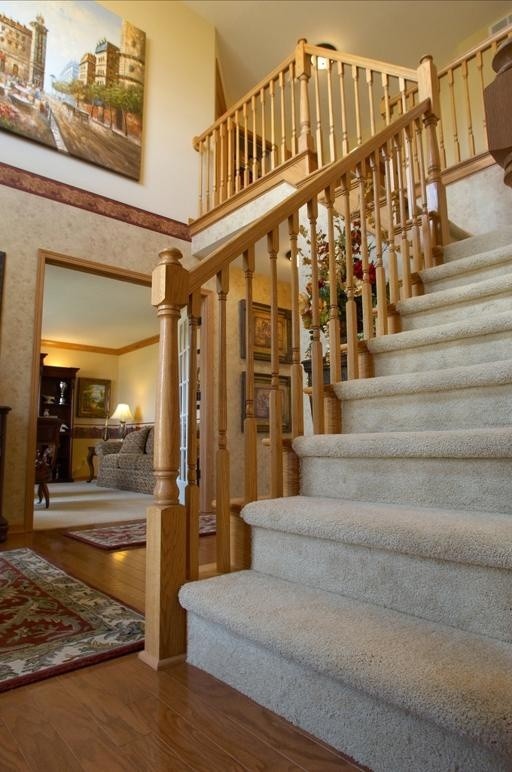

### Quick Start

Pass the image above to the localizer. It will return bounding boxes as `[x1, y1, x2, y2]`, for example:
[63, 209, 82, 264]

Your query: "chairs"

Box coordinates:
[33, 446, 55, 508]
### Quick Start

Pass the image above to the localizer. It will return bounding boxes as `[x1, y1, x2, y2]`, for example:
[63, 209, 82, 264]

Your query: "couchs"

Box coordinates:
[94, 432, 159, 494]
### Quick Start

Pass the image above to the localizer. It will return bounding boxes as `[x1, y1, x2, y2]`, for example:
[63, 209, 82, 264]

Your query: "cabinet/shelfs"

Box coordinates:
[37, 363, 81, 484]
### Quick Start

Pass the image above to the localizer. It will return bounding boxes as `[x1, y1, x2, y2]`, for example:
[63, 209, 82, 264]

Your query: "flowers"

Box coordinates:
[292, 216, 382, 334]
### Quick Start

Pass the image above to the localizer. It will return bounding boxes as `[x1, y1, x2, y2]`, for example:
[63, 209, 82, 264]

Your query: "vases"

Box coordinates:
[299, 354, 350, 415]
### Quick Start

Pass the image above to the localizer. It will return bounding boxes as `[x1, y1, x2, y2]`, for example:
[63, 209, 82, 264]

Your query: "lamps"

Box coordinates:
[109, 403, 136, 436]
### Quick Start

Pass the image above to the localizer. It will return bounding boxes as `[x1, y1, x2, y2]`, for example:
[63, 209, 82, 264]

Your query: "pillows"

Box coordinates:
[119, 427, 155, 456]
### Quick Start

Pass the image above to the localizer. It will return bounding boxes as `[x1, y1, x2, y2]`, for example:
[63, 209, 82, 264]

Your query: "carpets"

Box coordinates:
[58, 511, 218, 553]
[1, 543, 145, 691]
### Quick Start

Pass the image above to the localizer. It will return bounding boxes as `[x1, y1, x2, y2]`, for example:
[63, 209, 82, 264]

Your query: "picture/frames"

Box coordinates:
[78, 377, 112, 419]
[238, 299, 293, 434]
